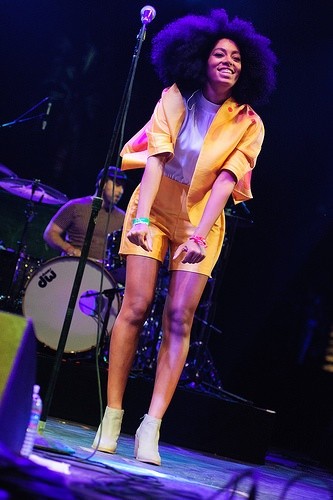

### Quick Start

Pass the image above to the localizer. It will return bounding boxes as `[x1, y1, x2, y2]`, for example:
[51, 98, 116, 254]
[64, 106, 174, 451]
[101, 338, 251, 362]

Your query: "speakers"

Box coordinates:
[0, 311, 38, 462]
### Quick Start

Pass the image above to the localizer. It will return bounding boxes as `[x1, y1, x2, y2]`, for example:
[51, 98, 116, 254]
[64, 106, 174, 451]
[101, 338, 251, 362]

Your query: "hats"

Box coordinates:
[98, 165, 131, 186]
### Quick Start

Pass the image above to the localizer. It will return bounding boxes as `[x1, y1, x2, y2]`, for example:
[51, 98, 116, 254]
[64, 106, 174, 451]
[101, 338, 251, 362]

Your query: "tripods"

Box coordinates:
[178, 274, 253, 406]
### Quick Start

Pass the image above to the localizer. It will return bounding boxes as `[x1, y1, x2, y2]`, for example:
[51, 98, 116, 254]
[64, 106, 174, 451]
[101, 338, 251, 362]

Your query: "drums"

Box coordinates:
[105, 226, 169, 279]
[22, 251, 123, 356]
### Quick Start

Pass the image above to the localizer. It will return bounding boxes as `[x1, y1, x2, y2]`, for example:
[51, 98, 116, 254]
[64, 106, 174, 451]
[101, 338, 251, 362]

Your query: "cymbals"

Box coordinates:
[0, 166, 69, 206]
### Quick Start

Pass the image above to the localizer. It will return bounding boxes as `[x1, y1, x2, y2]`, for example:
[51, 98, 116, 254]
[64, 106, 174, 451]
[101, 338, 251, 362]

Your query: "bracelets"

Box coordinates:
[133, 217, 150, 225]
[65, 245, 75, 252]
[188, 235, 208, 248]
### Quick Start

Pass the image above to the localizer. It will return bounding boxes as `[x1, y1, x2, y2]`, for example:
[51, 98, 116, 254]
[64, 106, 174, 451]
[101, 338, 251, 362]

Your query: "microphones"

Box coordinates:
[140, 5, 156, 24]
[42, 102, 51, 130]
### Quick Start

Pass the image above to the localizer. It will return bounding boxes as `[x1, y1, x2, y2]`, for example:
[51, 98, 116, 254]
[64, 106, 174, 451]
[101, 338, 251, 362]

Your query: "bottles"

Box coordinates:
[20, 385, 43, 458]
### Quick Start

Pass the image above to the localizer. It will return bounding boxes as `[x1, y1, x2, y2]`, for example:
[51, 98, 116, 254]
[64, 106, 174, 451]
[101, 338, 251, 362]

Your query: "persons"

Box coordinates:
[93, 8, 279, 466]
[42, 167, 128, 262]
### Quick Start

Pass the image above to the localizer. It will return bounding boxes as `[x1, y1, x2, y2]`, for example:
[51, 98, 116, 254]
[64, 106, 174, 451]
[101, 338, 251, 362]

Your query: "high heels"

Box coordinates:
[133, 413, 162, 466]
[91, 406, 123, 453]
